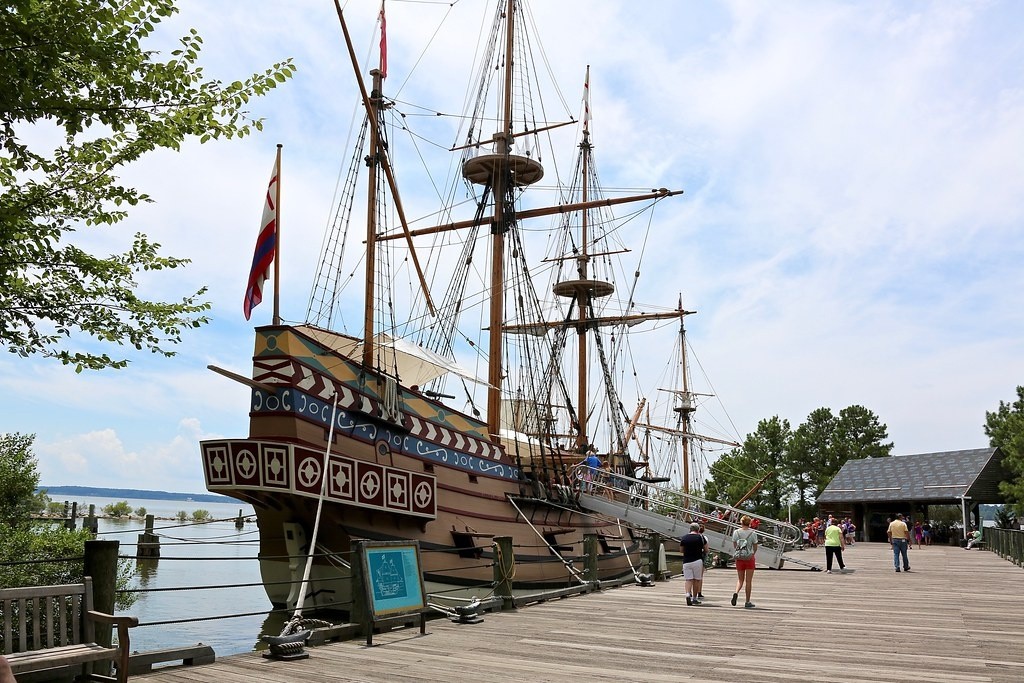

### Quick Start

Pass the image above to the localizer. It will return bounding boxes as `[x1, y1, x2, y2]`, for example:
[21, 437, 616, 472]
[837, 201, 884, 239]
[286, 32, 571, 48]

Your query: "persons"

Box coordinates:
[824, 520, 847, 574]
[798, 515, 856, 548]
[690, 504, 760, 533]
[887, 516, 932, 550]
[731, 516, 758, 607]
[575, 449, 614, 503]
[965, 524, 982, 549]
[680, 523, 709, 606]
[887, 513, 910, 572]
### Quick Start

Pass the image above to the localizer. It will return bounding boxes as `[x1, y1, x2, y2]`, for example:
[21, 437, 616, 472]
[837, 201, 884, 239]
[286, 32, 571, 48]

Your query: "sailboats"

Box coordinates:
[199, 1, 820, 611]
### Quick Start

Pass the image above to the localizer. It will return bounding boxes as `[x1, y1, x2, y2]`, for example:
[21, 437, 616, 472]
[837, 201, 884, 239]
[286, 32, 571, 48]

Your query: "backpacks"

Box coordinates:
[733, 531, 754, 559]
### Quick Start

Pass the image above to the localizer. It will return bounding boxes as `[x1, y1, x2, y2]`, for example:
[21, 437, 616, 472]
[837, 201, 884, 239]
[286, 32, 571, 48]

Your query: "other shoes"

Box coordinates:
[698, 595, 704, 597]
[731, 593, 738, 606]
[964, 547, 970, 550]
[745, 602, 755, 607]
[827, 570, 831, 574]
[686, 596, 692, 606]
[896, 569, 900, 572]
[909, 545, 912, 549]
[692, 600, 701, 604]
[690, 593, 692, 597]
[840, 567, 847, 571]
[904, 567, 910, 571]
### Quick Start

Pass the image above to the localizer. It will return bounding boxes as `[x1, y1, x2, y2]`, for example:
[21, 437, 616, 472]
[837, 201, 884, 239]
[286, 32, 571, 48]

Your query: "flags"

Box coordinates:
[243, 149, 281, 320]
[380, 11, 388, 75]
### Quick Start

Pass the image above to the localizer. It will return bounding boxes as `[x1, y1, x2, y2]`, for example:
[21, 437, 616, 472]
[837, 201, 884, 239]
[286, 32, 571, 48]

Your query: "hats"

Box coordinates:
[896, 513, 905, 519]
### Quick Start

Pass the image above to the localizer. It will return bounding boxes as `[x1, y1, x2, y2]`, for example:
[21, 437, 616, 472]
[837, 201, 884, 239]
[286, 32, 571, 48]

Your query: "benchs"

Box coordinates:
[0, 576, 139, 683]
[974, 541, 987, 550]
